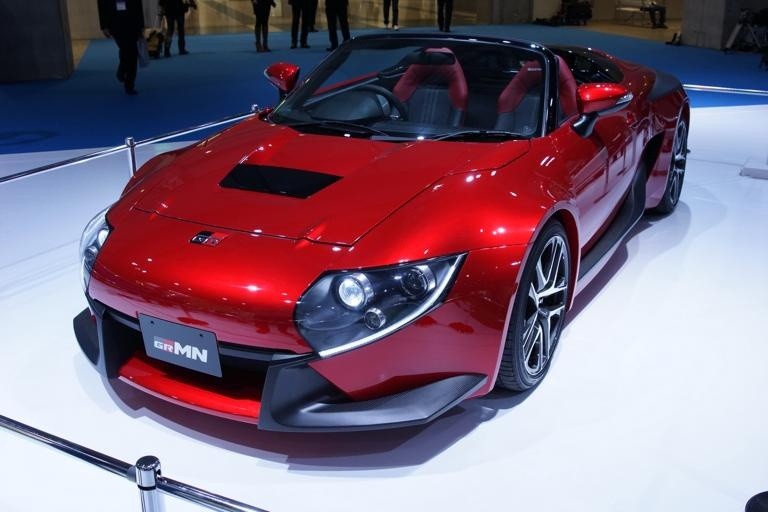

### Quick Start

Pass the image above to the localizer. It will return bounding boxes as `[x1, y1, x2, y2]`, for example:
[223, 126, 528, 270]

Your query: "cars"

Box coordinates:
[74, 29, 691, 436]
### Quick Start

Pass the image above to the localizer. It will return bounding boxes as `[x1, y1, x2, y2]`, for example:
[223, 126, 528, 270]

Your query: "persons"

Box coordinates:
[289, 1, 318, 49]
[97, 0, 143, 95]
[641, 0, 667, 28]
[325, 0, 350, 51]
[252, 0, 276, 52]
[437, 0, 453, 32]
[158, 0, 197, 57]
[383, 0, 399, 31]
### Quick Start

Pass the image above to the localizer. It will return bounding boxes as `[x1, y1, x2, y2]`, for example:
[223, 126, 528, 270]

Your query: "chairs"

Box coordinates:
[389, 47, 581, 138]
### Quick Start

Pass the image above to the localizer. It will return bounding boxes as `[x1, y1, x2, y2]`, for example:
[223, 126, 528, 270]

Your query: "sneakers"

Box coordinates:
[385, 23, 399, 30]
[290, 42, 310, 49]
[163, 45, 171, 57]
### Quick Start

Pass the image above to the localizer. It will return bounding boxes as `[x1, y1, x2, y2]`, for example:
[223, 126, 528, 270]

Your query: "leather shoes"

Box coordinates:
[117, 70, 136, 95]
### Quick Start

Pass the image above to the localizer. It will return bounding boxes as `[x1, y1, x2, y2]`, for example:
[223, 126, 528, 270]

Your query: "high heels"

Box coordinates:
[257, 46, 270, 52]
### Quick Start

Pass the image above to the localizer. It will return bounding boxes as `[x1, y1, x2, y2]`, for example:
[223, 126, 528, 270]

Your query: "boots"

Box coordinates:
[178, 40, 188, 55]
